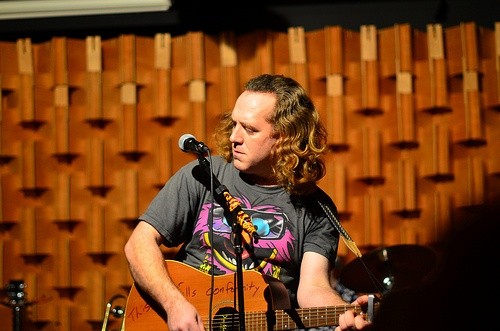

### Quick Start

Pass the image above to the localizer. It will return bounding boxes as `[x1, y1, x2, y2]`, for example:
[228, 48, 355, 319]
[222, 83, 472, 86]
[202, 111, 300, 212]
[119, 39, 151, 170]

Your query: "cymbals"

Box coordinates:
[340, 244, 436, 292]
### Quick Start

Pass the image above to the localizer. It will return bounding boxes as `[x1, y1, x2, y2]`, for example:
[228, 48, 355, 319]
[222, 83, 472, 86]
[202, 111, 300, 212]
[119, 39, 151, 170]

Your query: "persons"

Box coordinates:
[123, 73, 382, 331]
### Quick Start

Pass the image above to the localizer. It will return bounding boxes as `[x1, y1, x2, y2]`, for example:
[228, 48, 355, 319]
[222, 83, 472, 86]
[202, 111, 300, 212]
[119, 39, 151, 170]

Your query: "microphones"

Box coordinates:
[178, 134, 209, 152]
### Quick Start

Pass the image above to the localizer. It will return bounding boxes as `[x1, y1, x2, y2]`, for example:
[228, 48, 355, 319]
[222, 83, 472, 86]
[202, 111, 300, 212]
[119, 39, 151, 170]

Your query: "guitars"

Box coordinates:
[121, 259, 394, 330]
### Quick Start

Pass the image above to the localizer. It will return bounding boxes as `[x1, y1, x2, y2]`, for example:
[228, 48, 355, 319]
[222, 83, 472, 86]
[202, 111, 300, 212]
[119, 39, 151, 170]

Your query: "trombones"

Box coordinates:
[101, 293, 127, 331]
